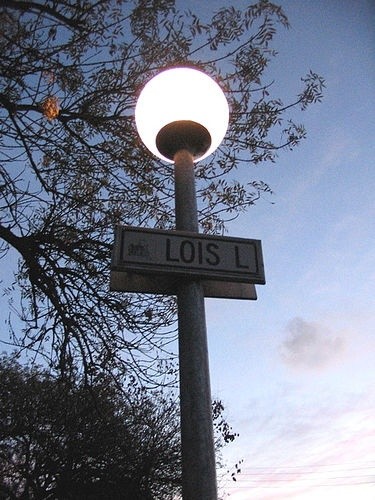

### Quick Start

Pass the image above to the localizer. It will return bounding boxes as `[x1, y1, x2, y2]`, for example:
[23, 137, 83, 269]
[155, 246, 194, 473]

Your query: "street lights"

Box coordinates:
[134, 65, 231, 499]
